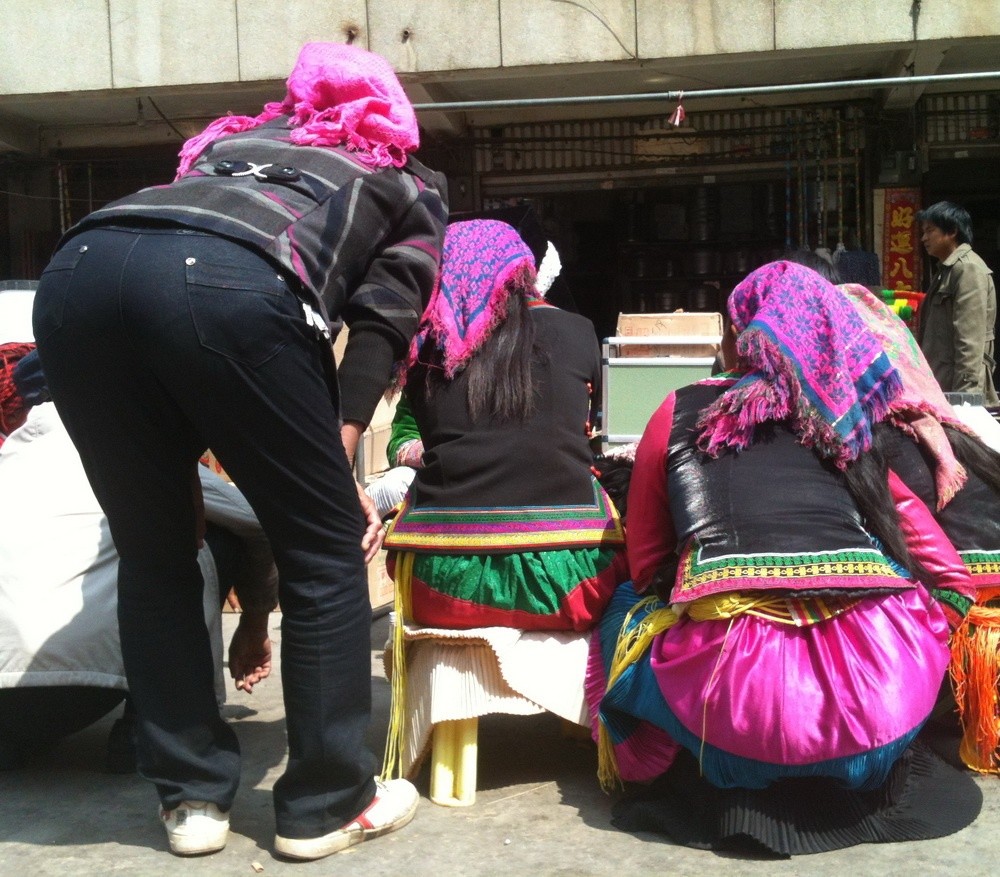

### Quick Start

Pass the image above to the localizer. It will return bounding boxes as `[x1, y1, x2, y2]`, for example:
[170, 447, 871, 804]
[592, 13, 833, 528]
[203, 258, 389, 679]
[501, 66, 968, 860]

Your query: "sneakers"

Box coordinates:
[275, 776, 419, 859]
[159, 800, 229, 854]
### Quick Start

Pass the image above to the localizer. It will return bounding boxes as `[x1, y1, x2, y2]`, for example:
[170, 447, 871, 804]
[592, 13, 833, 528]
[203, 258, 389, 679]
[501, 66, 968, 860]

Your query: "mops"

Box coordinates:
[831, 108, 849, 264]
[813, 107, 832, 278]
[837, 99, 880, 286]
[778, 117, 798, 261]
[793, 117, 825, 269]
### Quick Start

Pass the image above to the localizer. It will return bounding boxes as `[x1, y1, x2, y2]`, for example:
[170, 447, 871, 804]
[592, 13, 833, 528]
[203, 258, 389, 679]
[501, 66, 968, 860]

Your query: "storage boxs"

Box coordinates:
[617, 310, 722, 358]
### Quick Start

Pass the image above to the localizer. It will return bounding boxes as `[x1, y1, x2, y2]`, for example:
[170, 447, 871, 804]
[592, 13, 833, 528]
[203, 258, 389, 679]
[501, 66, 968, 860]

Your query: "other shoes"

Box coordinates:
[105, 718, 137, 773]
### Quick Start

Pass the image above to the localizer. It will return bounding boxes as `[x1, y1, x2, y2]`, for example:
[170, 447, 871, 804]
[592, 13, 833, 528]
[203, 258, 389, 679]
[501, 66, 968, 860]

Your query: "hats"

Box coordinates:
[13, 348, 52, 406]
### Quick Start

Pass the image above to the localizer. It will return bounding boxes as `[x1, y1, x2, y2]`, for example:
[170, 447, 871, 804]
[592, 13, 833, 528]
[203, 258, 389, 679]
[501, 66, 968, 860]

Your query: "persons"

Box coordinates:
[919, 201, 1000, 406]
[31, 41, 449, 861]
[1, 341, 30, 448]
[783, 250, 1000, 775]
[364, 219, 634, 634]
[1, 346, 278, 772]
[585, 259, 984, 861]
